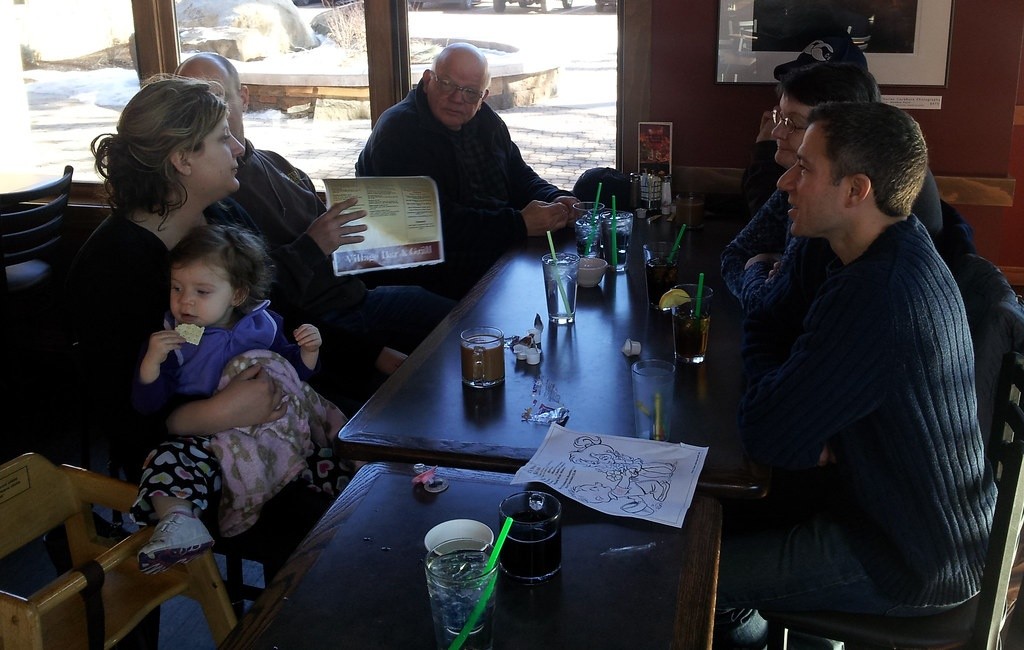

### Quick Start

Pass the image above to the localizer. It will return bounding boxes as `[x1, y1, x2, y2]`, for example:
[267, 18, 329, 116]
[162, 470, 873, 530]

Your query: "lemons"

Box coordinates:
[658, 289, 691, 309]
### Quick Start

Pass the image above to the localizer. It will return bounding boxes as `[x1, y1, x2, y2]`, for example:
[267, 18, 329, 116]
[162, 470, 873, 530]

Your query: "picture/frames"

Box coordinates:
[712, 1, 955, 92]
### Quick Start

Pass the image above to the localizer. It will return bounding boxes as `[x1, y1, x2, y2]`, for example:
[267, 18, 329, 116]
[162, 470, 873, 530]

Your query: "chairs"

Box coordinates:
[756, 348, 1024, 649]
[1, 165, 76, 455]
[0, 449, 238, 649]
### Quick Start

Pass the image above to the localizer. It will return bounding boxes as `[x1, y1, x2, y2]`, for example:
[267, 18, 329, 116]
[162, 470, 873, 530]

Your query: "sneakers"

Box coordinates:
[714, 605, 767, 644]
[138, 514, 215, 575]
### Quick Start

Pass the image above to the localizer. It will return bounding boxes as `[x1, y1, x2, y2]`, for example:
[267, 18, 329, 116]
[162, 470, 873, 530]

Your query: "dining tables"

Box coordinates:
[334, 224, 772, 505]
[219, 459, 725, 650]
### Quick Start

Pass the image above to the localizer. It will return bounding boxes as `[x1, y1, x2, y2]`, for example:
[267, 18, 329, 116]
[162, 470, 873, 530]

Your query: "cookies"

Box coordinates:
[174, 323, 205, 345]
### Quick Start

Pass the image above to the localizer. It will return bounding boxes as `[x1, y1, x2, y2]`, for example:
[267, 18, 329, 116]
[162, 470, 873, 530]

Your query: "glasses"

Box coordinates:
[431, 73, 483, 104]
[772, 110, 807, 133]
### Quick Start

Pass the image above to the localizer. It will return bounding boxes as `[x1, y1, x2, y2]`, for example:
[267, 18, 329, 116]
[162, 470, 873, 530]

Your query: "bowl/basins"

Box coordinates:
[578, 257, 607, 287]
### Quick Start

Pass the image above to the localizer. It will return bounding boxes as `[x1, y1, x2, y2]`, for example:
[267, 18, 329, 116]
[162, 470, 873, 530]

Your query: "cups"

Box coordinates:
[461, 325, 505, 388]
[631, 359, 675, 442]
[425, 538, 499, 650]
[643, 242, 681, 310]
[575, 200, 605, 258]
[498, 492, 563, 581]
[517, 346, 529, 360]
[424, 519, 494, 635]
[542, 252, 580, 324]
[636, 208, 646, 218]
[671, 284, 713, 363]
[676, 192, 705, 228]
[526, 347, 541, 364]
[599, 210, 633, 272]
[528, 329, 541, 343]
[662, 205, 670, 215]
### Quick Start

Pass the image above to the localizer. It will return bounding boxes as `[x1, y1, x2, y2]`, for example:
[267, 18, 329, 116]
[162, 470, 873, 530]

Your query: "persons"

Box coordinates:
[354, 46, 581, 305]
[69, 84, 366, 592]
[134, 225, 364, 575]
[711, 101, 999, 650]
[171, 51, 459, 420]
[742, 32, 943, 286]
[721, 64, 882, 429]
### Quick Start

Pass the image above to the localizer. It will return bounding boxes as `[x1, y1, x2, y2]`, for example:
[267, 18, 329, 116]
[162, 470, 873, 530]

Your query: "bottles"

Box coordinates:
[660, 175, 672, 207]
[629, 173, 640, 209]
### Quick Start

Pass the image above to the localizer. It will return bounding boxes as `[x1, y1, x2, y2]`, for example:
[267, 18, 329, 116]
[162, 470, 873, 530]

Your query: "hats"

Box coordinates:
[773, 30, 868, 86]
